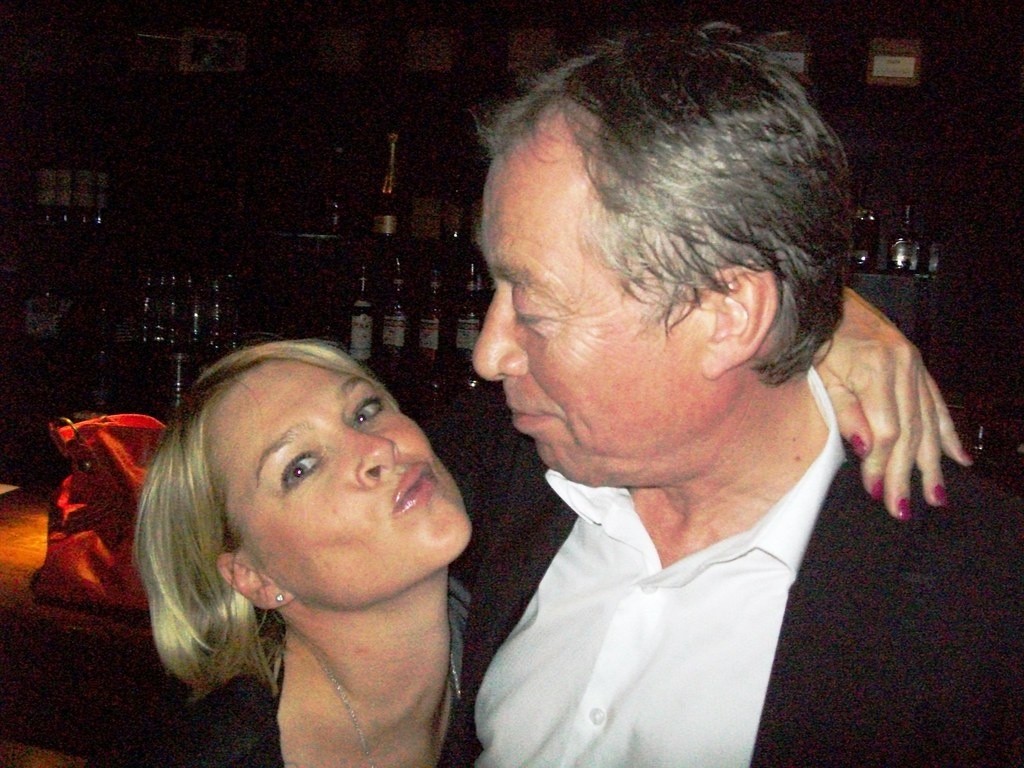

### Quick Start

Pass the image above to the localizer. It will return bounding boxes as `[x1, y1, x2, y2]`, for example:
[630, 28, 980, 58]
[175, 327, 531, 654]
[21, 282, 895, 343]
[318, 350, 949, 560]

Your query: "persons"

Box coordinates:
[108, 22, 1024, 768]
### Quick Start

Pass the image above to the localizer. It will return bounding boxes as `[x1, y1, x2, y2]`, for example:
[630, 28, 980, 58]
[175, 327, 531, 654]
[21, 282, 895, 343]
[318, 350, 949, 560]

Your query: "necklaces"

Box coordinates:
[287, 619, 464, 767]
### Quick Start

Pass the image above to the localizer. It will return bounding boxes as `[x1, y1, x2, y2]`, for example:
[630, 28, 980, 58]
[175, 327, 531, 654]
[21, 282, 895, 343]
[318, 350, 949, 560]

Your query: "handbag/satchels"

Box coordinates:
[29, 412, 167, 616]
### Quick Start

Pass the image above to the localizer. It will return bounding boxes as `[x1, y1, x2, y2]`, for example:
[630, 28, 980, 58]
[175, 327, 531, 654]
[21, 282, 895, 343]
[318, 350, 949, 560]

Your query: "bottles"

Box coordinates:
[372, 132, 403, 239]
[172, 352, 188, 410]
[891, 205, 912, 273]
[91, 261, 251, 348]
[34, 124, 112, 224]
[381, 257, 408, 359]
[452, 264, 483, 363]
[971, 427, 987, 466]
[419, 265, 445, 361]
[848, 180, 880, 275]
[409, 132, 483, 239]
[346, 266, 373, 363]
